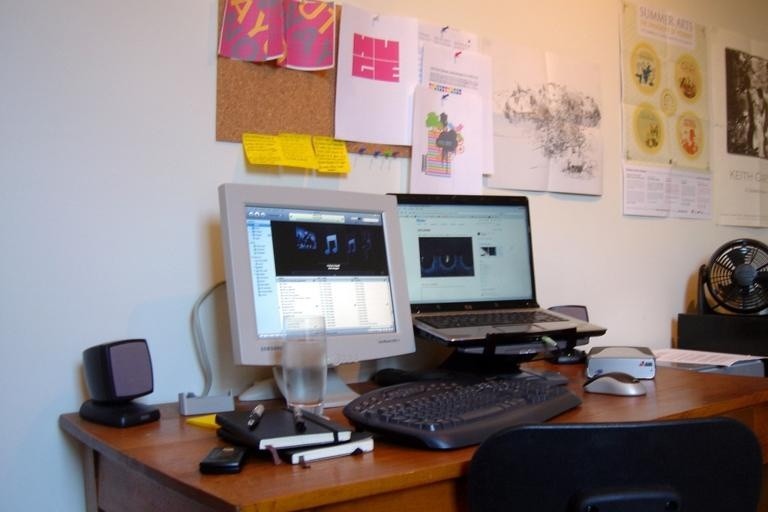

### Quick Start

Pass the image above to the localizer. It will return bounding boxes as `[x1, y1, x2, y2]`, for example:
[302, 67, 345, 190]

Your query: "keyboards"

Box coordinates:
[342, 368, 583, 451]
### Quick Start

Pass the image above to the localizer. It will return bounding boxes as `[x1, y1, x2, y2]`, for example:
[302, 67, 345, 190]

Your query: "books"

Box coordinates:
[214, 404, 353, 451]
[217, 427, 376, 467]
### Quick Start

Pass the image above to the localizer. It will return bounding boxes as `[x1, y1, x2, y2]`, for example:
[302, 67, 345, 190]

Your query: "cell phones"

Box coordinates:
[199, 447, 244, 474]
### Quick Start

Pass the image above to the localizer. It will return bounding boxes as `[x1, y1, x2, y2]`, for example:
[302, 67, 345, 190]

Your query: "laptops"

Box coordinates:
[385, 194, 607, 348]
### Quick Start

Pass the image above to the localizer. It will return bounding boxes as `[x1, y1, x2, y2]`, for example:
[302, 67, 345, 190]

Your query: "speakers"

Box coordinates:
[544, 305, 589, 364]
[79, 339, 160, 428]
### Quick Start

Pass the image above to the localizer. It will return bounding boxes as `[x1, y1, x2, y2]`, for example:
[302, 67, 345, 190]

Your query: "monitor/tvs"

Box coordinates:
[218, 183, 416, 409]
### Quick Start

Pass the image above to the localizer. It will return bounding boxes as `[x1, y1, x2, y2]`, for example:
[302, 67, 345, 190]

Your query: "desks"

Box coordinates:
[57, 346, 768, 512]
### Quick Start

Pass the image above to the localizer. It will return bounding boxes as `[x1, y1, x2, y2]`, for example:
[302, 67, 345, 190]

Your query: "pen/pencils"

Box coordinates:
[248, 403, 265, 427]
[294, 404, 307, 432]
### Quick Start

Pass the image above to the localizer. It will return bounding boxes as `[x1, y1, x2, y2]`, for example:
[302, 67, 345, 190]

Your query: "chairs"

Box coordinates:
[456, 416, 763, 511]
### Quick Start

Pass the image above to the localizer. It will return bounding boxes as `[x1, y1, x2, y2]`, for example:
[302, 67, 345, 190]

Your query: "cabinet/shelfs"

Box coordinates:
[676, 313, 768, 356]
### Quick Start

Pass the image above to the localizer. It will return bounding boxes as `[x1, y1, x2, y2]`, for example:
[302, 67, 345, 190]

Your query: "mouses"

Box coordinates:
[583, 372, 647, 397]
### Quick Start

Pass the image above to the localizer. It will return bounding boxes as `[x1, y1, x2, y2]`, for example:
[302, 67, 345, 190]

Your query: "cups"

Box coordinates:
[282, 314, 327, 416]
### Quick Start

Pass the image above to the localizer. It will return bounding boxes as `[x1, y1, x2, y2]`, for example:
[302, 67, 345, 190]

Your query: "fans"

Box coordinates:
[697, 239, 768, 317]
